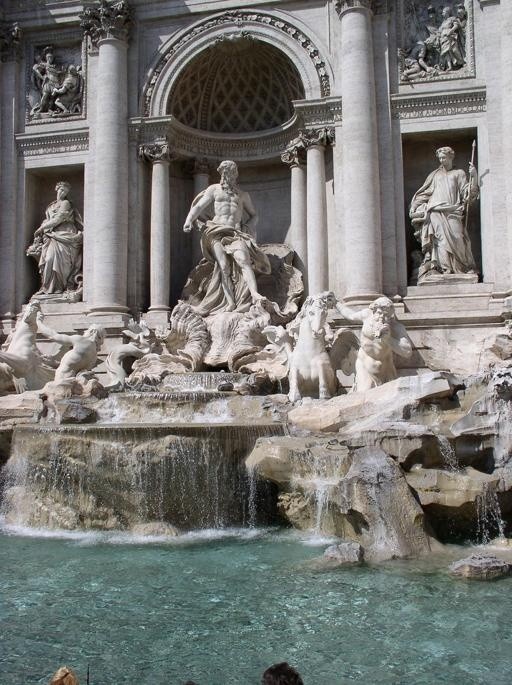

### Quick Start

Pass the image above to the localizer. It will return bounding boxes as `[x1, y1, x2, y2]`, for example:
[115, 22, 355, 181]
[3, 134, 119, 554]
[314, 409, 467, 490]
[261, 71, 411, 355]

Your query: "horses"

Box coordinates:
[0, 300, 57, 393]
[287, 294, 335, 403]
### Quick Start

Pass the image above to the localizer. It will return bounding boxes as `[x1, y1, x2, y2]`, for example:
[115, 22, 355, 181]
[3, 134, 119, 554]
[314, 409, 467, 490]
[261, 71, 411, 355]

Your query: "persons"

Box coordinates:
[183, 159, 267, 311]
[26, 181, 85, 296]
[394, 3, 470, 82]
[49, 665, 78, 684]
[37, 312, 106, 381]
[409, 148, 479, 285]
[330, 294, 412, 395]
[261, 663, 304, 684]
[31, 46, 81, 120]
